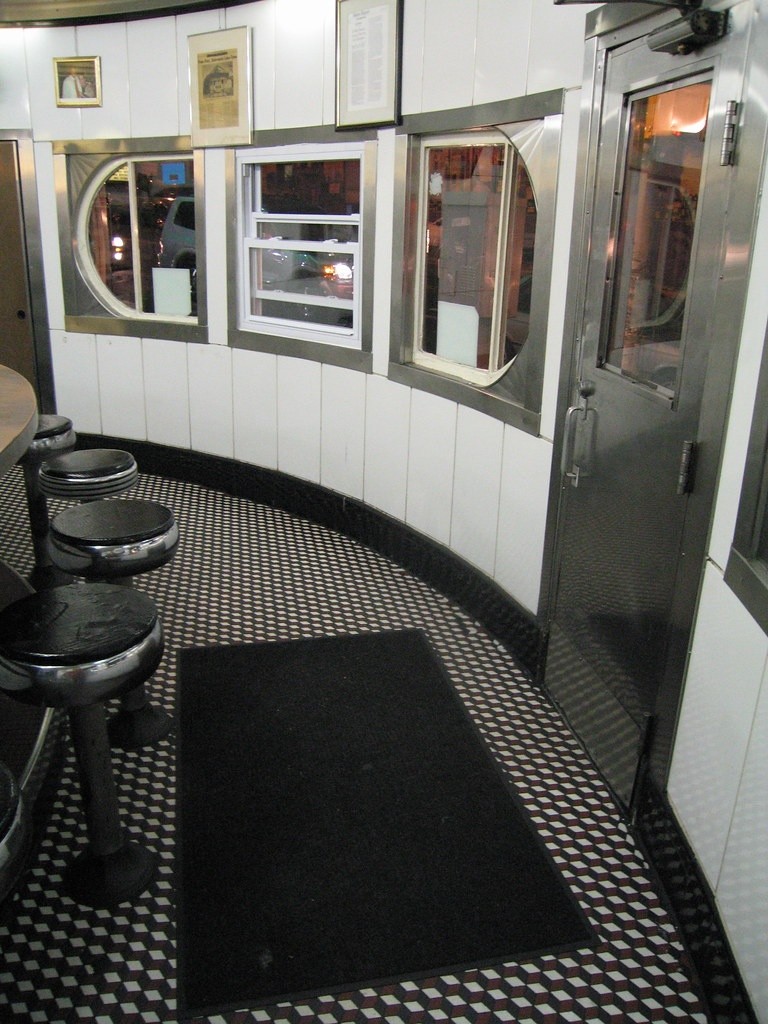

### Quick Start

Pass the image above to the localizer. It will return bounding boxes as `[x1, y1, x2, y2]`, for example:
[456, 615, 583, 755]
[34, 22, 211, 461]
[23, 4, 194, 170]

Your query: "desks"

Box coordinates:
[0, 364, 39, 609]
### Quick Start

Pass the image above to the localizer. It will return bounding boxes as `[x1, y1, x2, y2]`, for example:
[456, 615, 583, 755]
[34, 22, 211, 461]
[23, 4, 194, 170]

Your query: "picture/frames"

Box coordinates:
[53, 56, 103, 108]
[186, 25, 254, 149]
[334, 0, 405, 133]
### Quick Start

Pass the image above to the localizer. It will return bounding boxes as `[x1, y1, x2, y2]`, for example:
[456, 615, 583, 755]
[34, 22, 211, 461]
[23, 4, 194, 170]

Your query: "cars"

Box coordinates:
[60, 218, 124, 267]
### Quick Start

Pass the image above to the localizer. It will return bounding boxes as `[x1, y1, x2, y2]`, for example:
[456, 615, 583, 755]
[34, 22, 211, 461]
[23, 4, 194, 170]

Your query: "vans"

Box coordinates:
[157, 196, 355, 299]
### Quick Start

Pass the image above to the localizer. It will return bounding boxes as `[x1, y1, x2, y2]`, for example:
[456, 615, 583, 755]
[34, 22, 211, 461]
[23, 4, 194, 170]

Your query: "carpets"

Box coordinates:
[171, 627, 602, 1024]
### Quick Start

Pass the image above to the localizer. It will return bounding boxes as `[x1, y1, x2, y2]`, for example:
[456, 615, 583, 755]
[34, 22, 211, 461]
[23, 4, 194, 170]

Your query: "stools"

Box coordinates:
[38, 448, 138, 583]
[0, 583, 165, 910]
[46, 499, 180, 750]
[15, 413, 78, 590]
